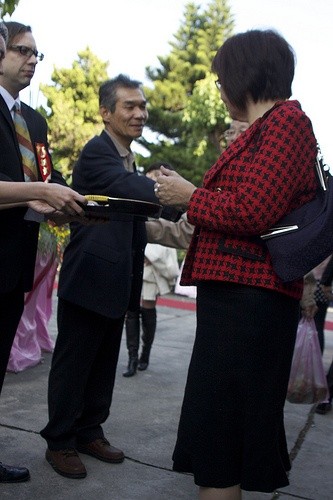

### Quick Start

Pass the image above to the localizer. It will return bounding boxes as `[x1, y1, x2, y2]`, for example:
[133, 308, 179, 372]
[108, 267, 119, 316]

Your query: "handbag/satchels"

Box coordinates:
[285, 317, 330, 403]
[258, 106, 333, 284]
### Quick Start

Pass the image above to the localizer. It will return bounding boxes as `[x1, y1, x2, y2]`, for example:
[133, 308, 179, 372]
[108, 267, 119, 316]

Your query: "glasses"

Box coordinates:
[215, 80, 221, 90]
[7, 44, 44, 61]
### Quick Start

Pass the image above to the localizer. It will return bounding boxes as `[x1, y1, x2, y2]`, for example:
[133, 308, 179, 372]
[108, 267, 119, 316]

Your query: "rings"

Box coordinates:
[157, 184, 160, 187]
[154, 188, 158, 192]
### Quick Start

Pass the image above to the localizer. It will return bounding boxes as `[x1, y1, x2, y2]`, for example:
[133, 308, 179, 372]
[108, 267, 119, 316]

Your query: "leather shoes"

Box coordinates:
[75, 436, 123, 463]
[0, 462, 31, 483]
[45, 447, 86, 479]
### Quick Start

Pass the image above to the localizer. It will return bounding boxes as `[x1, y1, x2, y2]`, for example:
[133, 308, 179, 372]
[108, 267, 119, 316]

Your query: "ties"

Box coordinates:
[12, 101, 38, 182]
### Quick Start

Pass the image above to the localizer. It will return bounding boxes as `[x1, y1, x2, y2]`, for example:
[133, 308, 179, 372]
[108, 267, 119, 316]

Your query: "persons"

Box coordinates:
[123, 164, 179, 378]
[39, 75, 182, 480]
[0, 22, 111, 482]
[298, 255, 333, 414]
[153, 30, 318, 500]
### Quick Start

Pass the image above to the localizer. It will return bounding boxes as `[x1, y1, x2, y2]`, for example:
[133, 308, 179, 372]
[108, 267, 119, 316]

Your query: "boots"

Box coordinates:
[122, 305, 140, 377]
[138, 307, 157, 370]
